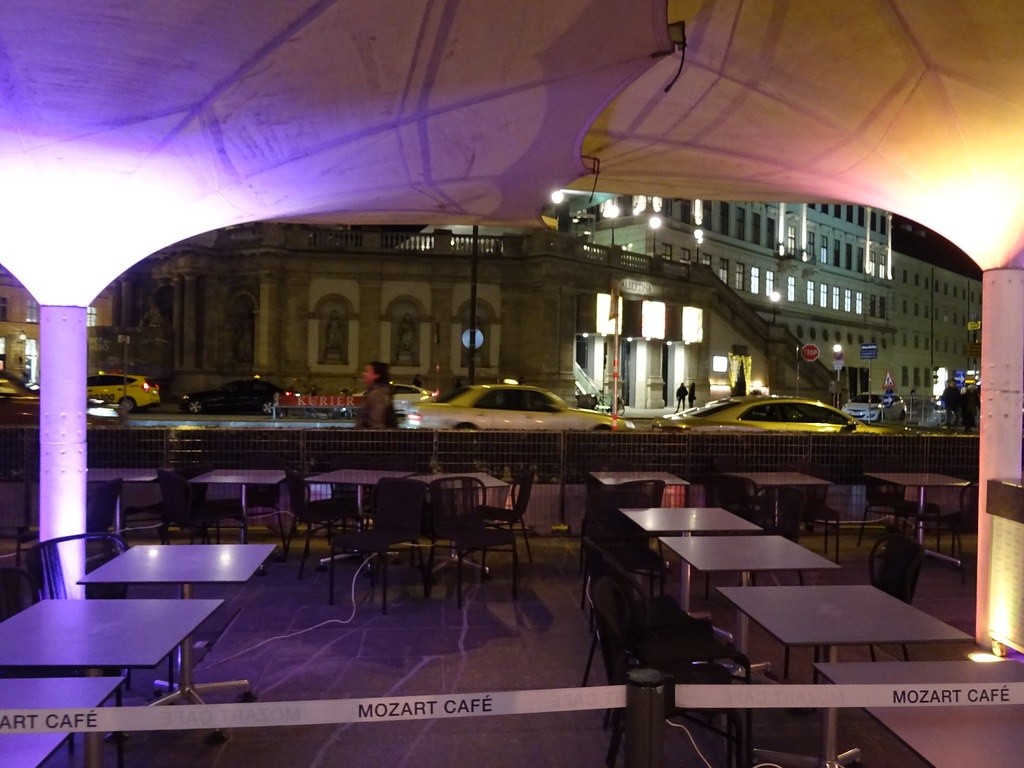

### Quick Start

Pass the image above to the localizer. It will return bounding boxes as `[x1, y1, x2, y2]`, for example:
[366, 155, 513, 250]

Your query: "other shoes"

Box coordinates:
[947, 427, 952, 432]
[676, 410, 678, 413]
[965, 428, 972, 434]
[953, 428, 961, 434]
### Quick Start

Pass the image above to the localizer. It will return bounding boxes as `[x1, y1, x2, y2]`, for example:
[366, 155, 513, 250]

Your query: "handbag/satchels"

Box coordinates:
[692, 396, 696, 400]
[361, 403, 398, 428]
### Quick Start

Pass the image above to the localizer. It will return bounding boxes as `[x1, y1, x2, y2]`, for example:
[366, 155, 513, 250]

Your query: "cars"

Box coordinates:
[399, 384, 635, 432]
[87, 373, 161, 414]
[842, 392, 907, 423]
[351, 383, 439, 417]
[180, 379, 288, 415]
[651, 395, 918, 434]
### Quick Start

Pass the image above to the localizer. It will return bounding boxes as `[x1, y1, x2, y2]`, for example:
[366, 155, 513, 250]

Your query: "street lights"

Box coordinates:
[649, 216, 661, 257]
[770, 292, 780, 322]
[694, 229, 705, 263]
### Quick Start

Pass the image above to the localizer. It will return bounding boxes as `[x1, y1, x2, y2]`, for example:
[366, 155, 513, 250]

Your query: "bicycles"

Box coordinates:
[591, 389, 625, 417]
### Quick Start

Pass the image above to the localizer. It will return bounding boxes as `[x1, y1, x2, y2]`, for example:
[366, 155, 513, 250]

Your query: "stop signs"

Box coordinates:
[801, 343, 821, 363]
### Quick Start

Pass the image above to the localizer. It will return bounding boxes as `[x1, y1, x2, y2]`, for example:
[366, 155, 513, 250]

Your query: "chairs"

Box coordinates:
[0, 461, 981, 768]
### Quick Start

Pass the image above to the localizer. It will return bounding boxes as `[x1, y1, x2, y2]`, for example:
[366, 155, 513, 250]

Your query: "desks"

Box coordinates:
[812, 659, 1024, 768]
[188, 470, 296, 577]
[588, 471, 690, 486]
[77, 545, 278, 743]
[0, 598, 225, 768]
[720, 471, 834, 528]
[303, 468, 416, 579]
[0, 676, 127, 768]
[87, 468, 159, 563]
[716, 585, 975, 768]
[658, 536, 842, 728]
[863, 473, 979, 567]
[405, 472, 510, 582]
[617, 507, 764, 644]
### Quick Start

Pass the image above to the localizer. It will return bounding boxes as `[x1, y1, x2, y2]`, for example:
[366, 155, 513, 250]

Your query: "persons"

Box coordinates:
[412, 373, 422, 388]
[961, 384, 980, 435]
[353, 361, 400, 430]
[941, 379, 962, 434]
[676, 383, 688, 413]
[688, 382, 696, 408]
